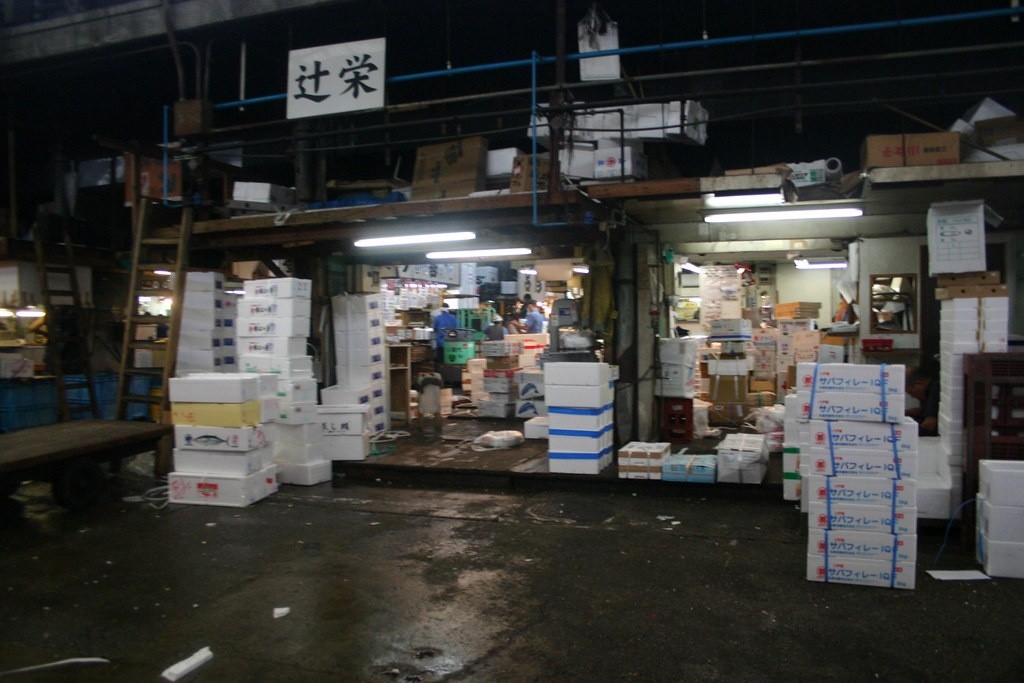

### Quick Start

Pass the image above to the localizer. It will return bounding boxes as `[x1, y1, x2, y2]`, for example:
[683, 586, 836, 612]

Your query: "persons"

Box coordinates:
[905, 365, 941, 436]
[484, 293, 543, 340]
[432, 303, 457, 373]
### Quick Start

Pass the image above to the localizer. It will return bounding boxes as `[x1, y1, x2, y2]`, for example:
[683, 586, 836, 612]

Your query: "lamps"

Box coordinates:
[696, 206, 865, 226]
[519, 265, 538, 275]
[573, 262, 589, 274]
[794, 255, 849, 270]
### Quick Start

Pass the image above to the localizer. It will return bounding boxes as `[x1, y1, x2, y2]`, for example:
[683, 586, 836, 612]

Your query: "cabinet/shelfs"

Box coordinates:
[386, 340, 414, 429]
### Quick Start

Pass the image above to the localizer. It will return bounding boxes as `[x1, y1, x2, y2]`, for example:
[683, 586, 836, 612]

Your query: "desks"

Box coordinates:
[0, 415, 175, 527]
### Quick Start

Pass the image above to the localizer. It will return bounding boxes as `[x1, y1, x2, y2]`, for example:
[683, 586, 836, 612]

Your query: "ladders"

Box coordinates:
[31, 209, 99, 419]
[114, 198, 194, 425]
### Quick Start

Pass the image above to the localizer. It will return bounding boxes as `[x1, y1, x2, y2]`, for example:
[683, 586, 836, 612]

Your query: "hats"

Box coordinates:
[492, 315, 504, 322]
[522, 299, 536, 308]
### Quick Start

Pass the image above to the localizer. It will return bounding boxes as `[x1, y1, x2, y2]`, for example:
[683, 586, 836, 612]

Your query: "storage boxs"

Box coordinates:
[0, 95, 1024, 601]
